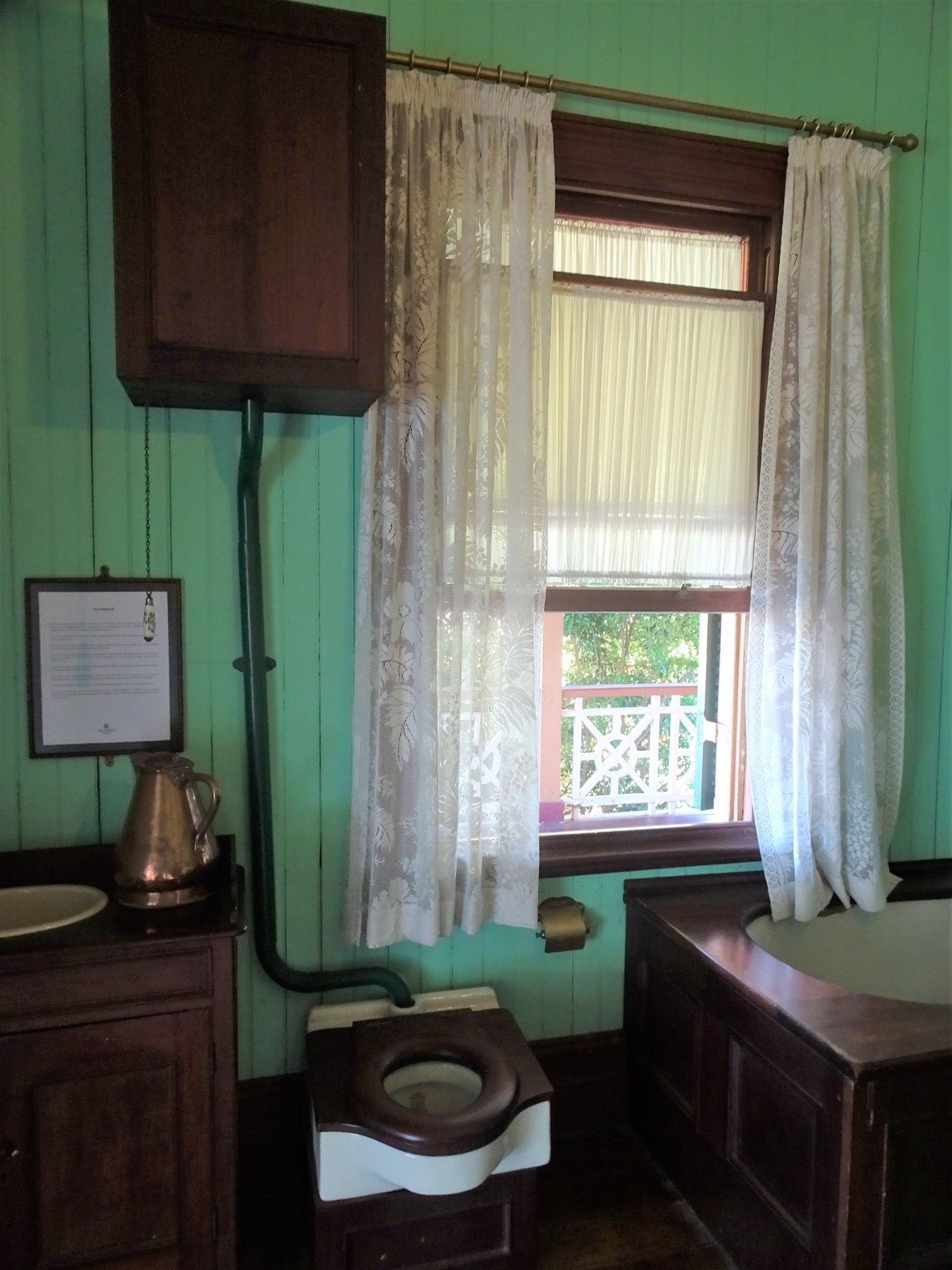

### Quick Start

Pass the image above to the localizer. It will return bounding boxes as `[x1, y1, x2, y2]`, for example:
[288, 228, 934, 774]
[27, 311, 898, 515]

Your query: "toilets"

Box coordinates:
[304, 984, 555, 1205]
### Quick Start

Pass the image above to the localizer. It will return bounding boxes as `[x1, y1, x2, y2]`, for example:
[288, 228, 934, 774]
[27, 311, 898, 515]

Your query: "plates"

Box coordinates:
[0, 885, 108, 938]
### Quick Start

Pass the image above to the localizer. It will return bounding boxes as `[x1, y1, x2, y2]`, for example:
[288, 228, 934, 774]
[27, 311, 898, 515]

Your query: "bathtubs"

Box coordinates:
[622, 859, 950, 1268]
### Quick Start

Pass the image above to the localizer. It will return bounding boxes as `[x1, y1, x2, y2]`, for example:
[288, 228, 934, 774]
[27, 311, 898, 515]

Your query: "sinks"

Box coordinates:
[0, 883, 108, 938]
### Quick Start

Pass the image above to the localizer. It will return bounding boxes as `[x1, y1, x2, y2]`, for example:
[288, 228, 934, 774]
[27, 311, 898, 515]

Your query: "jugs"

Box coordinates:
[109, 750, 223, 910]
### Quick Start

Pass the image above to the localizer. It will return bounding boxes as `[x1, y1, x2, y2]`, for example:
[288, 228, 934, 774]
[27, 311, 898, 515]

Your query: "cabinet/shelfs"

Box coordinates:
[0, 936, 242, 1270]
